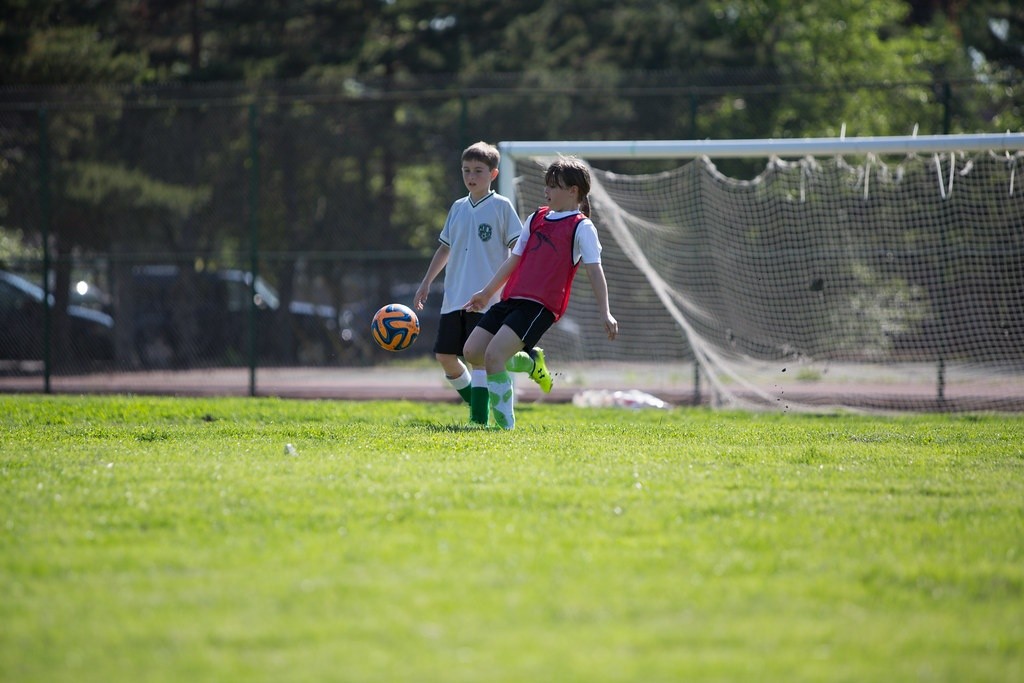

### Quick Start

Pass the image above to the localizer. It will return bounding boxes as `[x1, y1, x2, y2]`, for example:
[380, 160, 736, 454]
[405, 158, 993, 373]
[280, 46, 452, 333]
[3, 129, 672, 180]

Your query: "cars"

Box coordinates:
[0, 270, 116, 365]
[121, 266, 338, 368]
[332, 283, 579, 360]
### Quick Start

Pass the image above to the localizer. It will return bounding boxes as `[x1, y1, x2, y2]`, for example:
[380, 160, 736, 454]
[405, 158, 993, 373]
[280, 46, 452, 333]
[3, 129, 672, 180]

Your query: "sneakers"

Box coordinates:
[529, 346, 553, 393]
[464, 420, 486, 429]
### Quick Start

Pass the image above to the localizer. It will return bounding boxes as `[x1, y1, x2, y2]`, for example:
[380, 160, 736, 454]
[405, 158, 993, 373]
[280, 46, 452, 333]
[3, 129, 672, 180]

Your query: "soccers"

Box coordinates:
[371, 303, 420, 352]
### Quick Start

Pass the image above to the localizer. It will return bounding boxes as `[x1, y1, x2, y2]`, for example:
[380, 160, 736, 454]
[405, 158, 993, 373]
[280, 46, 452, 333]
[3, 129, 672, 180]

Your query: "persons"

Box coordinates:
[414, 143, 553, 428]
[461, 151, 618, 432]
